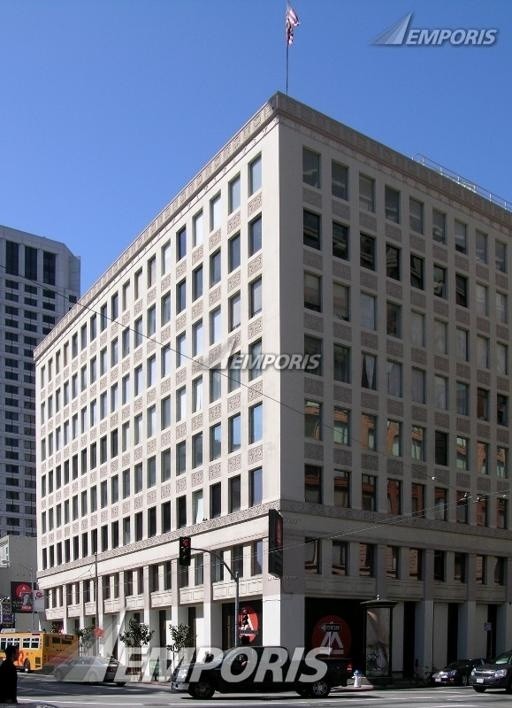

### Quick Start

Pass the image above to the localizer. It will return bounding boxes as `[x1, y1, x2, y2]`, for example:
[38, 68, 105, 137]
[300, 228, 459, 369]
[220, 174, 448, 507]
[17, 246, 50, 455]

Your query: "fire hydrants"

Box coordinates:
[352, 668, 362, 688]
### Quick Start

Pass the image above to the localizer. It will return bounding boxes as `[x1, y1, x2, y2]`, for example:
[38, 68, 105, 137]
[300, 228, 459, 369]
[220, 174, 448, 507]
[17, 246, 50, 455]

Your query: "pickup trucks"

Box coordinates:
[172, 644, 354, 699]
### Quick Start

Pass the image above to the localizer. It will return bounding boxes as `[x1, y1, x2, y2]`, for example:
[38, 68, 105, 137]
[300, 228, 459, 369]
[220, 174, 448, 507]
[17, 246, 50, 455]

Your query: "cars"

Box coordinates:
[54, 655, 132, 685]
[432, 657, 492, 686]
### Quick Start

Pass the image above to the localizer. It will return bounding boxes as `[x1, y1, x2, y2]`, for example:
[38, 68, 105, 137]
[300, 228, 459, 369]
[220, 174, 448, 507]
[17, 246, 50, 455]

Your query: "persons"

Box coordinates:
[239, 607, 254, 637]
[1, 645, 22, 704]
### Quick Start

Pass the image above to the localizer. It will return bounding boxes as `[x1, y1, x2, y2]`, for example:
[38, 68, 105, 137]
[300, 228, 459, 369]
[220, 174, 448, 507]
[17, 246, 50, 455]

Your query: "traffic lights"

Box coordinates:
[179, 535, 191, 565]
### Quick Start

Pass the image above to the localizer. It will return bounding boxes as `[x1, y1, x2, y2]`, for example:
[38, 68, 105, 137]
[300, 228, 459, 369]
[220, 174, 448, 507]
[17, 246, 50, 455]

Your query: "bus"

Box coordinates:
[0, 627, 79, 673]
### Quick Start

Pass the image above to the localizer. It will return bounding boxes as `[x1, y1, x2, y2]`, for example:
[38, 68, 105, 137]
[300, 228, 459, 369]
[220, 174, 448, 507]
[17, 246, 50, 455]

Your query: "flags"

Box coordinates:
[285, 5, 300, 47]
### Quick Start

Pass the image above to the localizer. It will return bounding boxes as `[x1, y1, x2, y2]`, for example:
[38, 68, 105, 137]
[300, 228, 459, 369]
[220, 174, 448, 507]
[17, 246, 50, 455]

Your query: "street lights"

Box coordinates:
[1, 559, 36, 632]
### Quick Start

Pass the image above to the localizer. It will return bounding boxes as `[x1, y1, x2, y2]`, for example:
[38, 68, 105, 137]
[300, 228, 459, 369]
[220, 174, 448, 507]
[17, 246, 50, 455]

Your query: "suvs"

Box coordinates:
[470, 649, 512, 693]
